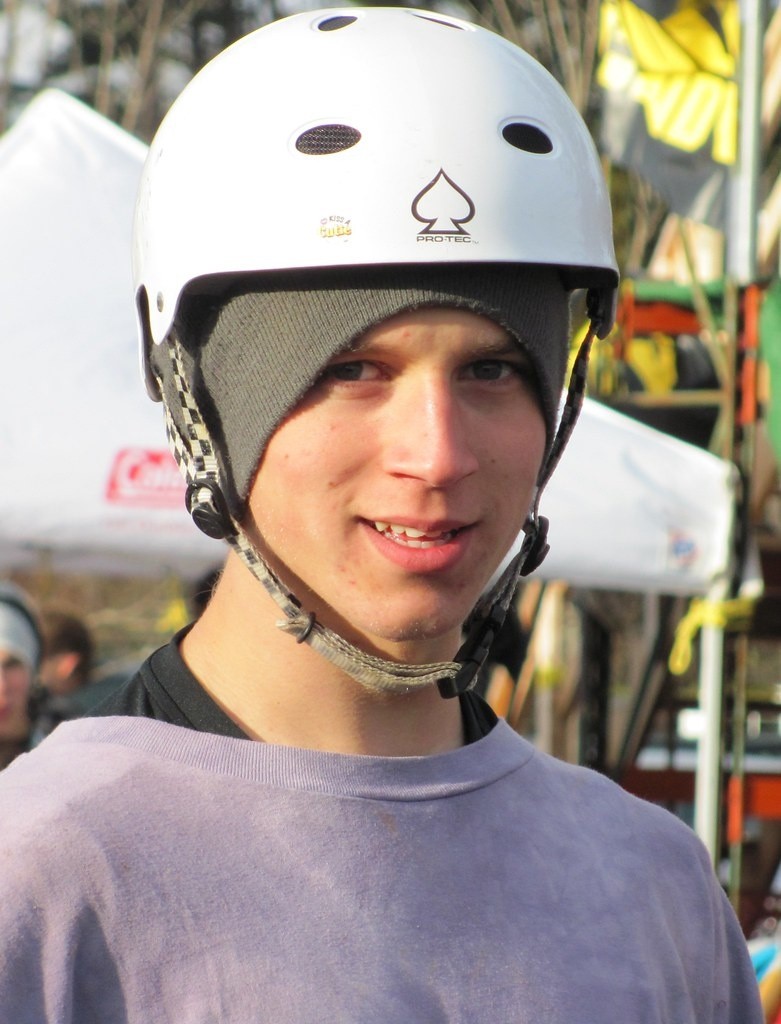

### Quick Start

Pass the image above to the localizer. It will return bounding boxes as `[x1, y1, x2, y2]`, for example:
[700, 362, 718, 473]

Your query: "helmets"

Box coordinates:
[134, 7, 619, 402]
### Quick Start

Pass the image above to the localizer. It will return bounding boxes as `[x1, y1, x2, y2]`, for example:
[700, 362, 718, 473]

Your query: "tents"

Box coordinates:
[0, 89, 741, 870]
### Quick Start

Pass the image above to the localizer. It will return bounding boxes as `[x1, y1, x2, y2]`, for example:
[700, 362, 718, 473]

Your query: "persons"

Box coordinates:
[0, 573, 223, 770]
[0, 7, 762, 1023]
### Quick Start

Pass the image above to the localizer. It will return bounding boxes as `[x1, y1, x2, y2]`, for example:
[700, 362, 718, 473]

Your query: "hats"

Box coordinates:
[0, 577, 48, 672]
[186, 264, 572, 522]
